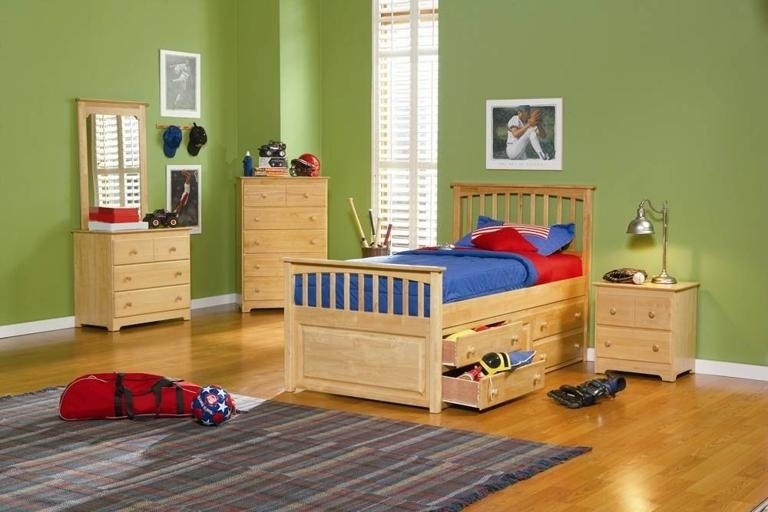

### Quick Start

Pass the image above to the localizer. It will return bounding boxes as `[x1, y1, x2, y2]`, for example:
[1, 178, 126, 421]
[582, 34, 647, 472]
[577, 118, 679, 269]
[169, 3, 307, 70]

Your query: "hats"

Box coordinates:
[163, 126, 182, 157]
[188, 122, 207, 156]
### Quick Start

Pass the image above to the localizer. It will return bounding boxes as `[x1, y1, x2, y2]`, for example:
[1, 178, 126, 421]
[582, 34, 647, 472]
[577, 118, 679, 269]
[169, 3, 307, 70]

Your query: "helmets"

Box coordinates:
[289, 154, 320, 176]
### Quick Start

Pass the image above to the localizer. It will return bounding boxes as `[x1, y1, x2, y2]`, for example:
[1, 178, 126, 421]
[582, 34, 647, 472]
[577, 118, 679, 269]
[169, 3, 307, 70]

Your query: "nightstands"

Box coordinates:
[594, 279, 699, 382]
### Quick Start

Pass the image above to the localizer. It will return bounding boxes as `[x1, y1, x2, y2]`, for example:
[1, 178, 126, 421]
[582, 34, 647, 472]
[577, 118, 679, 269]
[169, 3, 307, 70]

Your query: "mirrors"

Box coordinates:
[75, 96, 148, 229]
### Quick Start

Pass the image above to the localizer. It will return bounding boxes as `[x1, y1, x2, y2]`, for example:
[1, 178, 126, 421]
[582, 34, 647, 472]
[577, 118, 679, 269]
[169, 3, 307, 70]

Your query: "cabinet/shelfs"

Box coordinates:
[238, 174, 331, 315]
[69, 228, 192, 331]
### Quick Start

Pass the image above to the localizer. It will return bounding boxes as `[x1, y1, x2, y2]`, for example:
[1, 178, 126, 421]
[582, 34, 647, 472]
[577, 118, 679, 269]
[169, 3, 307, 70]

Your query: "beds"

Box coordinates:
[280, 181, 597, 418]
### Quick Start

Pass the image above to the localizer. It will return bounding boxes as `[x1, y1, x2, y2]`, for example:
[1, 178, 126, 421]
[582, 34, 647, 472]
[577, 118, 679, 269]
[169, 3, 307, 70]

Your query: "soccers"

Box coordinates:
[191, 385, 232, 426]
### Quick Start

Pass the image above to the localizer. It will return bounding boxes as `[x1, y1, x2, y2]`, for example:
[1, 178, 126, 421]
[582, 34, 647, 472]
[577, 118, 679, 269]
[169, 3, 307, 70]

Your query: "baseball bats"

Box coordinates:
[348, 198, 369, 248]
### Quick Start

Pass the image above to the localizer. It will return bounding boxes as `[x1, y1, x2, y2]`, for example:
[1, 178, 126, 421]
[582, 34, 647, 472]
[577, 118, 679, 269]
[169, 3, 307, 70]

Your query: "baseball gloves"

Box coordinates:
[528, 110, 545, 127]
[603, 268, 648, 283]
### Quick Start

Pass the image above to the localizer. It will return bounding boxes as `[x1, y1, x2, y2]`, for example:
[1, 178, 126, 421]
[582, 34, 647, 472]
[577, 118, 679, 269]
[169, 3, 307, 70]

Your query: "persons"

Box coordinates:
[170, 58, 194, 108]
[505, 106, 555, 160]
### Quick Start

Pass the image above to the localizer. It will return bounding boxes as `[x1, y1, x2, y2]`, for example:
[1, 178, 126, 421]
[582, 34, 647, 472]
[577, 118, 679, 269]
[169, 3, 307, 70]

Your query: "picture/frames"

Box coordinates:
[484, 97, 563, 171]
[159, 48, 201, 118]
[166, 164, 202, 235]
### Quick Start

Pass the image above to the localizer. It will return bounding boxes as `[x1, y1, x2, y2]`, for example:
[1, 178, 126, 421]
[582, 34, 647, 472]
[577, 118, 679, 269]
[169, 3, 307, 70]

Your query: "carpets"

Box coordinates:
[1, 381, 593, 511]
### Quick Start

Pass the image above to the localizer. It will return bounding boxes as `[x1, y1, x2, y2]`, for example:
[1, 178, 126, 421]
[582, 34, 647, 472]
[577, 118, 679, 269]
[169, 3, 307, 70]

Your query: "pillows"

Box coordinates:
[453, 214, 576, 255]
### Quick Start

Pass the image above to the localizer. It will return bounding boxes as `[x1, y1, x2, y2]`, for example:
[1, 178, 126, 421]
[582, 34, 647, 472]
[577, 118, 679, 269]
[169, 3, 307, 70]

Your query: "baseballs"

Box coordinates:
[632, 272, 645, 284]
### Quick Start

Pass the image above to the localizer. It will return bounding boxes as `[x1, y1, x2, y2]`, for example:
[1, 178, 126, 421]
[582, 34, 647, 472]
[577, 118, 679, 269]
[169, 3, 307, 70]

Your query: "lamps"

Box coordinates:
[627, 199, 677, 285]
